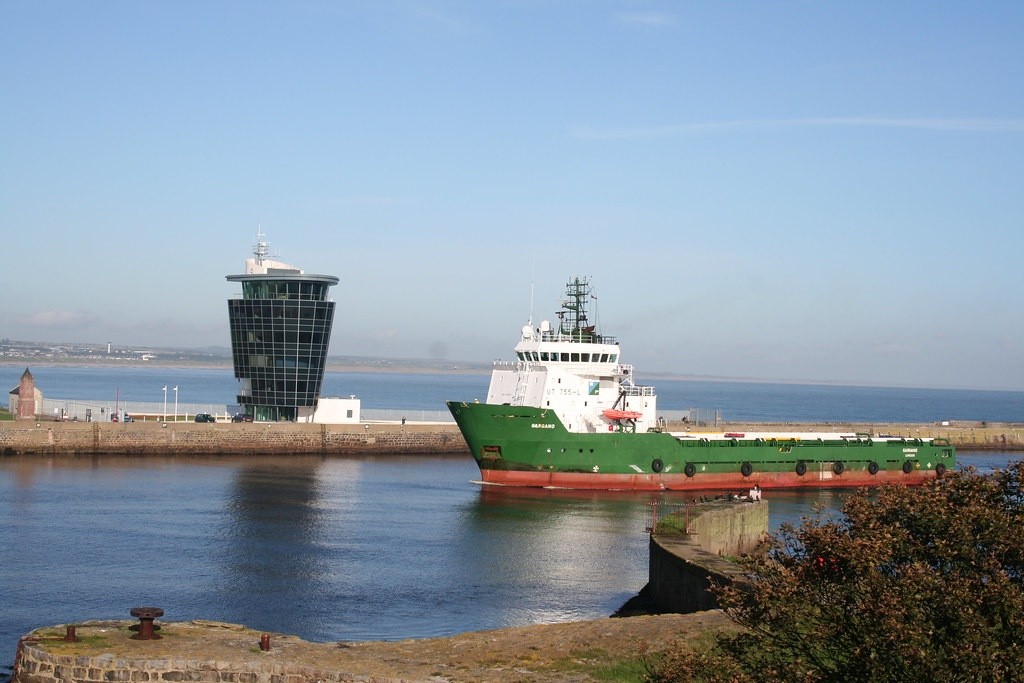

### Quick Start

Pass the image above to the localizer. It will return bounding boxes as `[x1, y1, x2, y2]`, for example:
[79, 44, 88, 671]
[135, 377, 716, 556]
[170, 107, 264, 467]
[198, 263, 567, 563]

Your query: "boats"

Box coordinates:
[602, 408, 643, 420]
[445, 274, 956, 492]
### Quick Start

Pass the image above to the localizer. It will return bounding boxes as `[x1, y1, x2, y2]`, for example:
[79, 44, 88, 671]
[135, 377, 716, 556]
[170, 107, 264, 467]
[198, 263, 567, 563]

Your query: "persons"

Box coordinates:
[402, 416, 406, 425]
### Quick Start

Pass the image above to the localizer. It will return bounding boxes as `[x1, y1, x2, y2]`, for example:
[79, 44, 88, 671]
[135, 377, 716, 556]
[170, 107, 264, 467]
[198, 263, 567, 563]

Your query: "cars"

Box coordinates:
[231, 414, 253, 423]
[195, 414, 215, 423]
[111, 412, 133, 422]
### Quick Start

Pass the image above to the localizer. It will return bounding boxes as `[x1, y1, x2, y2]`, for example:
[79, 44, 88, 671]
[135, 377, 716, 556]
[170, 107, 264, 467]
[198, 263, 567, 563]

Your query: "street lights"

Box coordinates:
[172, 385, 179, 421]
[162, 385, 167, 421]
